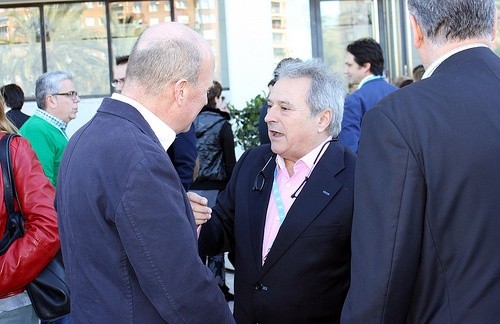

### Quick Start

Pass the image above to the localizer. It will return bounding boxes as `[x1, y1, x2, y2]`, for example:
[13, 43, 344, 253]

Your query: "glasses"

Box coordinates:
[111, 77, 124, 88]
[51, 91, 78, 101]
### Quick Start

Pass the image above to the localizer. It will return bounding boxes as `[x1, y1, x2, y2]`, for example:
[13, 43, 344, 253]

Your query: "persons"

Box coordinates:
[198, 57, 358, 324]
[18, 71, 80, 324]
[0, 84, 33, 133]
[55, 23, 236, 324]
[0, 88, 60, 324]
[192, 80, 236, 303]
[220, 38, 426, 159]
[340, 0, 500, 324]
[111, 53, 197, 194]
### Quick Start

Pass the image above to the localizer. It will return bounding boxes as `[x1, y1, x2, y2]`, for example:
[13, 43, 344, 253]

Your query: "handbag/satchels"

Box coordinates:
[0, 134, 71, 322]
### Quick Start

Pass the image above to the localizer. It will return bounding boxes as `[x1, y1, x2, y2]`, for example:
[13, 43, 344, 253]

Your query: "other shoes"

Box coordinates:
[224, 291, 234, 302]
